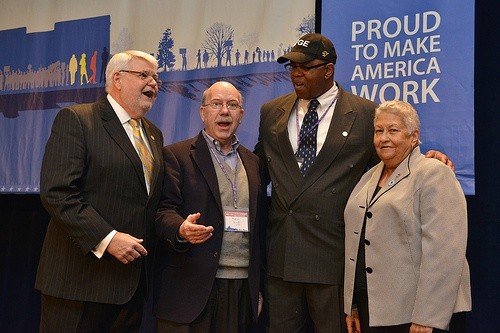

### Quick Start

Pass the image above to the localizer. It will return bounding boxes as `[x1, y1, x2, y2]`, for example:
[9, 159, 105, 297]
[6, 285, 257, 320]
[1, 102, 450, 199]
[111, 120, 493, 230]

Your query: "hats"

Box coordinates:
[276, 33, 337, 65]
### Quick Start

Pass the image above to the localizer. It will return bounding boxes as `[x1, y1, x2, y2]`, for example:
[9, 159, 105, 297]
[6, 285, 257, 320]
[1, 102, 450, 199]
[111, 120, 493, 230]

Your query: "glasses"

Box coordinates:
[203, 100, 242, 110]
[284, 62, 329, 73]
[118, 69, 162, 88]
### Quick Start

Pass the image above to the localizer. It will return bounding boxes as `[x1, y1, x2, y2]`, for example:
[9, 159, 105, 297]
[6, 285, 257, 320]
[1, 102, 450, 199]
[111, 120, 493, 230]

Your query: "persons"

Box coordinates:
[246, 32, 458, 333]
[342, 101, 472, 333]
[33, 50, 164, 333]
[151, 80, 264, 333]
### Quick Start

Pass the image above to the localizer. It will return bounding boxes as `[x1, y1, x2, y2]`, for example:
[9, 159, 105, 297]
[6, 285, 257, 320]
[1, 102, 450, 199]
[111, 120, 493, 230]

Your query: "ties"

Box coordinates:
[297, 98, 319, 177]
[128, 118, 154, 193]
